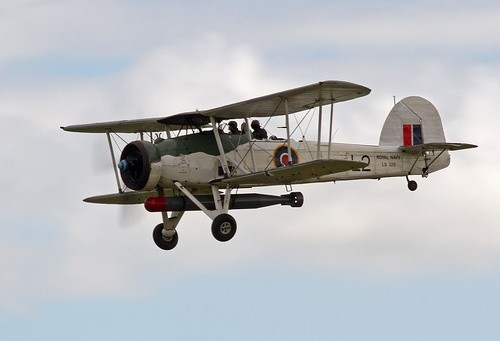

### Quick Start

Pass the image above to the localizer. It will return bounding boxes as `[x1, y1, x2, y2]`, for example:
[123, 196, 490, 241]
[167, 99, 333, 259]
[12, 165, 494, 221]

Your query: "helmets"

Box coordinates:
[241, 122, 247, 130]
[228, 121, 237, 130]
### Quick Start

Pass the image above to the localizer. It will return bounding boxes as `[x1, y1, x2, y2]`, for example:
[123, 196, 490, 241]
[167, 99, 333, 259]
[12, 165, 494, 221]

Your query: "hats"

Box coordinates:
[251, 120, 259, 126]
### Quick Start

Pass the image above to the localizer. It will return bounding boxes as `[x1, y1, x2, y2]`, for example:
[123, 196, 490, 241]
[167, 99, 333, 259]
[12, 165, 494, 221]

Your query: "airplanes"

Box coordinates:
[59, 79, 479, 251]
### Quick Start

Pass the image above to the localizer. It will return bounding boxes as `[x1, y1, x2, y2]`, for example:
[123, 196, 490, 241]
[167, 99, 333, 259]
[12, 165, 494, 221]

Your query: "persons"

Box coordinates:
[241, 123, 251, 135]
[251, 120, 267, 139]
[227, 121, 241, 134]
